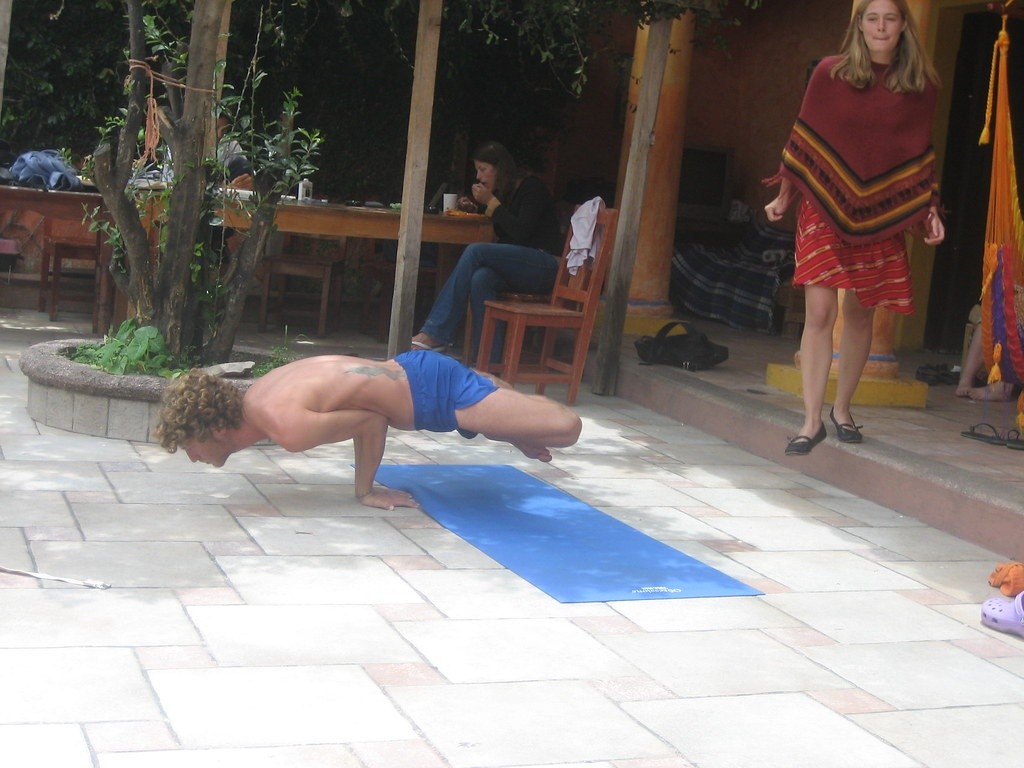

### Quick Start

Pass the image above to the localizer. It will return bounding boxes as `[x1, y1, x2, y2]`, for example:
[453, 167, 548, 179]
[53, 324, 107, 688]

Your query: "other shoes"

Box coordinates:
[938, 371, 984, 387]
[915, 363, 939, 385]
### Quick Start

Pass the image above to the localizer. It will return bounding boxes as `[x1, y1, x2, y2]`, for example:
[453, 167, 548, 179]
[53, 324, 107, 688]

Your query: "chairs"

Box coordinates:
[257, 191, 346, 339]
[48, 224, 100, 322]
[497, 206, 572, 364]
[357, 191, 436, 345]
[475, 204, 617, 407]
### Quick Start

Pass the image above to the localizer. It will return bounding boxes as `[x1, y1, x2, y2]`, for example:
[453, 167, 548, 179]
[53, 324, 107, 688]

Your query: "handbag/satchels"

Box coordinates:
[7, 149, 83, 193]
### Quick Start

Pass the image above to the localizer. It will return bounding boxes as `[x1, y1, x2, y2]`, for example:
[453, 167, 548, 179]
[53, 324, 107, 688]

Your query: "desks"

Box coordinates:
[126, 188, 495, 364]
[675, 219, 754, 260]
[0, 183, 120, 337]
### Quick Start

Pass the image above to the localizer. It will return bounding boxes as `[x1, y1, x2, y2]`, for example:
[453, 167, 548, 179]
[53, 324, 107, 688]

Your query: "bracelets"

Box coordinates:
[359, 491, 373, 498]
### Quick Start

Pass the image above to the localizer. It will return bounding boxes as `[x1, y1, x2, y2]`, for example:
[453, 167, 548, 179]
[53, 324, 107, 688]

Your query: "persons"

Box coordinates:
[411, 140, 594, 362]
[143, 107, 254, 278]
[155, 348, 582, 511]
[762, 0, 946, 457]
[956, 321, 1014, 402]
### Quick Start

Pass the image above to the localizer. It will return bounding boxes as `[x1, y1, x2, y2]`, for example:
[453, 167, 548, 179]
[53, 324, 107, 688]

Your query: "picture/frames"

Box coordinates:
[611, 52, 633, 130]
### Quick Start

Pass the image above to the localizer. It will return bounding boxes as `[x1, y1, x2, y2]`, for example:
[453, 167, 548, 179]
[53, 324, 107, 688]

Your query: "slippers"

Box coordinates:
[411, 337, 447, 352]
[1006, 429, 1024, 450]
[961, 423, 1006, 445]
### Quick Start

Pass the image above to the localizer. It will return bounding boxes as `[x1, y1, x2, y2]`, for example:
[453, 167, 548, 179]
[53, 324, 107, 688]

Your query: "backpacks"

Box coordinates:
[633, 321, 729, 371]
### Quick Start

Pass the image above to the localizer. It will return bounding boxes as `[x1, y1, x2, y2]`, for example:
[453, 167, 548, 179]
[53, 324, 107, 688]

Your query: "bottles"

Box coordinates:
[297, 179, 313, 201]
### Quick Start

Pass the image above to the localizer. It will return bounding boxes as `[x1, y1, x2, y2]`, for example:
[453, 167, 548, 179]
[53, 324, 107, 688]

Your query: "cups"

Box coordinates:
[443, 194, 458, 212]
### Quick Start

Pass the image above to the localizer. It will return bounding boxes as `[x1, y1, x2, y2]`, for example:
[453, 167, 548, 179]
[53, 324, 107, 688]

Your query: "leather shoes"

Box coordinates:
[785, 422, 827, 455]
[829, 405, 865, 443]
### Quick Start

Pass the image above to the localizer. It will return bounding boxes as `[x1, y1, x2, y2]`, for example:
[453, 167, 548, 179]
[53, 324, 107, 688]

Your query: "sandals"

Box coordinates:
[981, 591, 1024, 638]
